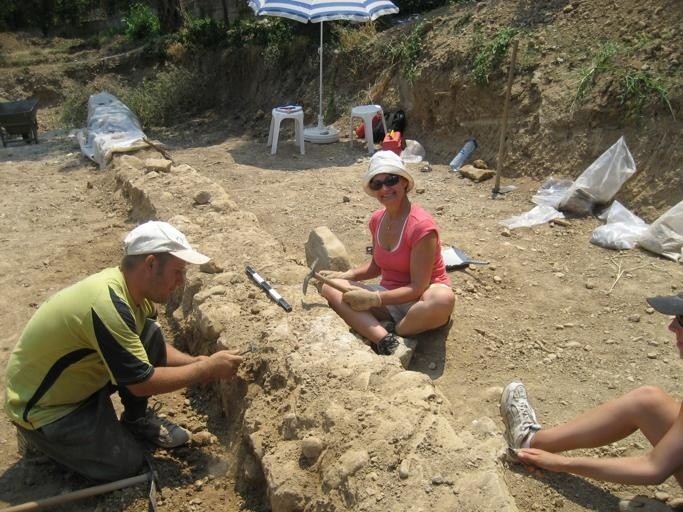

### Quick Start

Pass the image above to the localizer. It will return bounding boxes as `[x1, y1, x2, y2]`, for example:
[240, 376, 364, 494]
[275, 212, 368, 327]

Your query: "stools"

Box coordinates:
[349, 105, 388, 153]
[267, 109, 305, 156]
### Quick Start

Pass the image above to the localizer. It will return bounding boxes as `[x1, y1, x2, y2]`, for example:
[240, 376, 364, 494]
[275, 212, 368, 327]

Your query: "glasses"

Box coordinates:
[369, 175, 400, 191]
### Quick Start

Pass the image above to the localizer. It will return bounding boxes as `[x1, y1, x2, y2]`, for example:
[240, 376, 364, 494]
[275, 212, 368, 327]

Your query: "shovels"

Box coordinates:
[440, 245, 490, 268]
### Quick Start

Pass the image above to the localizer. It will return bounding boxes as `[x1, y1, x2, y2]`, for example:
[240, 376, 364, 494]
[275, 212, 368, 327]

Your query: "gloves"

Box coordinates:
[319, 268, 356, 282]
[343, 286, 382, 312]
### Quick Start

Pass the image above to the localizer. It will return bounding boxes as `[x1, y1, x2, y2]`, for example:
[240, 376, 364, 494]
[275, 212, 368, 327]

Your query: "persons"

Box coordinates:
[318, 149, 455, 354]
[4, 219, 244, 484]
[498, 290, 683, 491]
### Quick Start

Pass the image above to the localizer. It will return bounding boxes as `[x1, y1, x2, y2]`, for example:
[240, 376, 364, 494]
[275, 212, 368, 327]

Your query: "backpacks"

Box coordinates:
[373, 109, 406, 144]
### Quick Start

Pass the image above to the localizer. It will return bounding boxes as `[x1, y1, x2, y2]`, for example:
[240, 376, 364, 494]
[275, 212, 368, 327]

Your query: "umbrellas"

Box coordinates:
[245, 0, 399, 144]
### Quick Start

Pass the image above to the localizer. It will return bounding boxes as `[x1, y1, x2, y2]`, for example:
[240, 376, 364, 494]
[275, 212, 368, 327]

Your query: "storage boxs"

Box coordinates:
[383, 127, 402, 157]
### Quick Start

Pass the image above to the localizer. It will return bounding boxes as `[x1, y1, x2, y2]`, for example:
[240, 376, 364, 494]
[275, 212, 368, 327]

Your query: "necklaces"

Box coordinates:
[387, 211, 399, 231]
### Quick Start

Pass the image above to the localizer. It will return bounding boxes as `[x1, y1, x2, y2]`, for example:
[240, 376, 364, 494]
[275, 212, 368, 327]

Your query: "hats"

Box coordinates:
[123, 220, 211, 264]
[361, 151, 414, 198]
[646, 292, 682, 315]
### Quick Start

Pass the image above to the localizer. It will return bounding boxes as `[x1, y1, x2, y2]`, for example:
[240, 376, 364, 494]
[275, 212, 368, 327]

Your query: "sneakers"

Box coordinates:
[121, 401, 192, 449]
[362, 319, 418, 369]
[500, 381, 542, 464]
[15, 427, 50, 464]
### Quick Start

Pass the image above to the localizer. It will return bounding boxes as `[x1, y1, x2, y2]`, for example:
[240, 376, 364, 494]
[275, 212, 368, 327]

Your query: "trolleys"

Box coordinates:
[0, 96, 42, 145]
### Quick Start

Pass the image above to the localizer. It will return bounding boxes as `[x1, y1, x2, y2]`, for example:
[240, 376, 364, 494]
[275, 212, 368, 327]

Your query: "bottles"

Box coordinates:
[448, 139, 479, 174]
